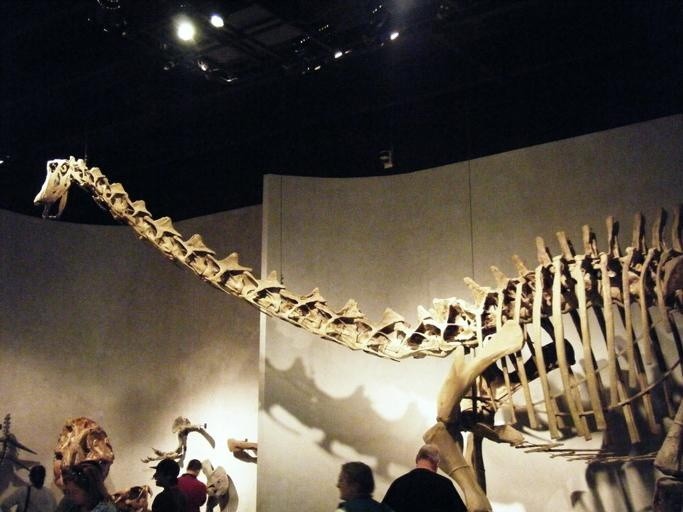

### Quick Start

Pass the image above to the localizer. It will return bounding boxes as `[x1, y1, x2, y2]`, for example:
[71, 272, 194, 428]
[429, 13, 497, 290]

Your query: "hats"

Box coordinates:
[150, 458, 180, 472]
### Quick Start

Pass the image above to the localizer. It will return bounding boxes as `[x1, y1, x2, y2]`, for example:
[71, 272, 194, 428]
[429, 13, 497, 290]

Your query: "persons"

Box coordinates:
[175, 458, 206, 512]
[333, 461, 381, 512]
[379, 444, 470, 512]
[0, 462, 58, 511]
[59, 464, 117, 512]
[148, 458, 197, 512]
